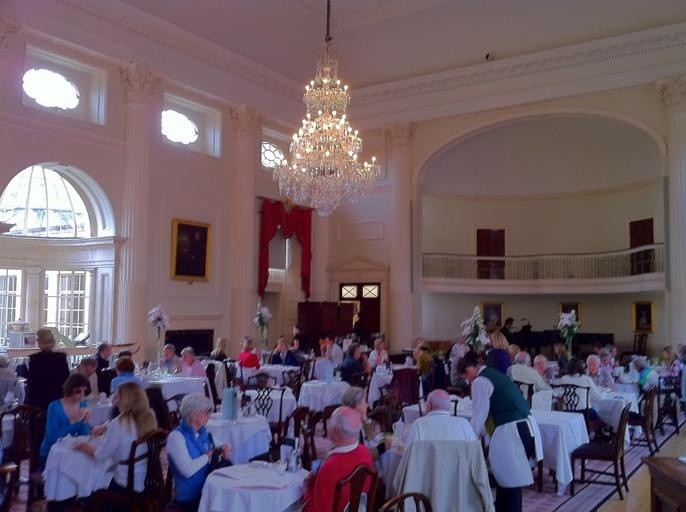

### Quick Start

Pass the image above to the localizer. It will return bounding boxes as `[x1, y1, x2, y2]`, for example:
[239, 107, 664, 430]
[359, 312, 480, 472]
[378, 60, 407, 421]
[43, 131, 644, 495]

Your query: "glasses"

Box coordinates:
[72, 388, 88, 394]
[194, 406, 213, 417]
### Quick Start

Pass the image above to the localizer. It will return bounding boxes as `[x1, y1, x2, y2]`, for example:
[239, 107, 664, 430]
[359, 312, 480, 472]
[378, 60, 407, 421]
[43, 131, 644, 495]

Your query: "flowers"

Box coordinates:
[252, 302, 272, 334]
[461, 306, 491, 345]
[557, 309, 576, 337]
[147, 303, 169, 342]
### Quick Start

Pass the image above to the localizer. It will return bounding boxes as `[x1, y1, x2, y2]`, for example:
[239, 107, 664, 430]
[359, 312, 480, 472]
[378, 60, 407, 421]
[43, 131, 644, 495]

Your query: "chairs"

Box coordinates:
[620, 333, 649, 367]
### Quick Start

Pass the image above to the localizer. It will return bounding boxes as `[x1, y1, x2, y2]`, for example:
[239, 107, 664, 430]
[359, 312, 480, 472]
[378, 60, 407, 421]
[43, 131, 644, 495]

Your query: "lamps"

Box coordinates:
[271, 0, 377, 217]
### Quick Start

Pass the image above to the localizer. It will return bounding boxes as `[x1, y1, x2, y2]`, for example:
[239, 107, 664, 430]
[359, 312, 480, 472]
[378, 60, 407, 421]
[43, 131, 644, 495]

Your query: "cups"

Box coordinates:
[392, 421, 404, 432]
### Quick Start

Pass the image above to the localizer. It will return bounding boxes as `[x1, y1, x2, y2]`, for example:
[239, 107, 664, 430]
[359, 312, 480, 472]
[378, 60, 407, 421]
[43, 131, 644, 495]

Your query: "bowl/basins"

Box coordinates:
[272, 460, 287, 473]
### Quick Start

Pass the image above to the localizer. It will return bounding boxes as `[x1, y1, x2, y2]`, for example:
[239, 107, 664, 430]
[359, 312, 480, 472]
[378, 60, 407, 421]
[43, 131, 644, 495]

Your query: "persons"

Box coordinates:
[1, 318, 686, 512]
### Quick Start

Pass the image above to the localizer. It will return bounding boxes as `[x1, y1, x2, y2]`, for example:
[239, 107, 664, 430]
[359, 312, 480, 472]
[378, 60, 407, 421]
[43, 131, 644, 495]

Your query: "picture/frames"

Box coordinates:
[171, 217, 211, 282]
[481, 302, 504, 330]
[632, 301, 655, 334]
[559, 302, 581, 327]
